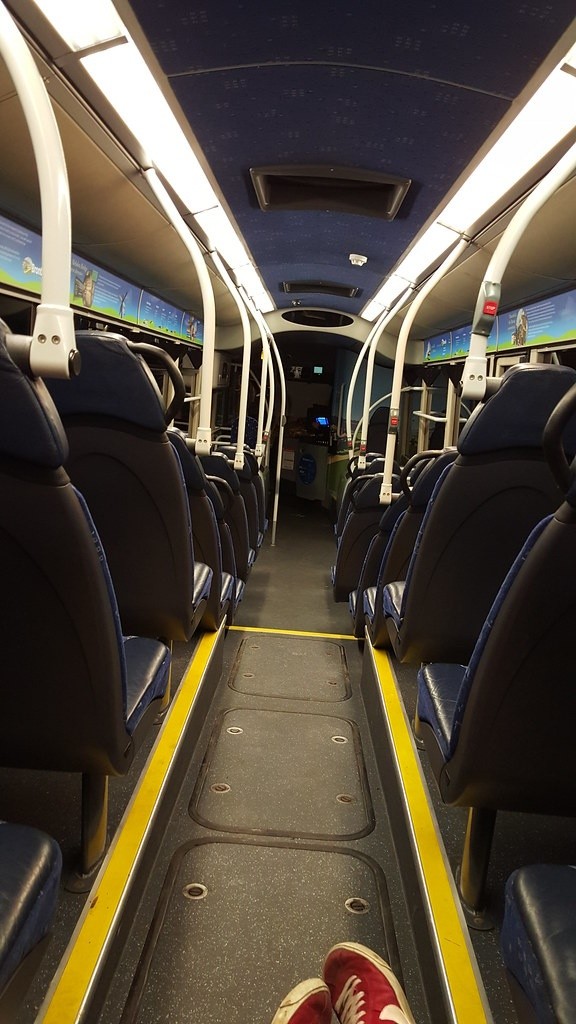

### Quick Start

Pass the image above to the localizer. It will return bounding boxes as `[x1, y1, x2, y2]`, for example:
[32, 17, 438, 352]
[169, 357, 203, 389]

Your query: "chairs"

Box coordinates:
[330, 360, 576, 1024]
[0, 319, 272, 1024]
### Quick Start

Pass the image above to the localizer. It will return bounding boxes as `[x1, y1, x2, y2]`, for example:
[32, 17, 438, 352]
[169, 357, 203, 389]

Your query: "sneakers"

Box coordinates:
[271, 978, 332, 1024]
[323, 942, 416, 1024]
